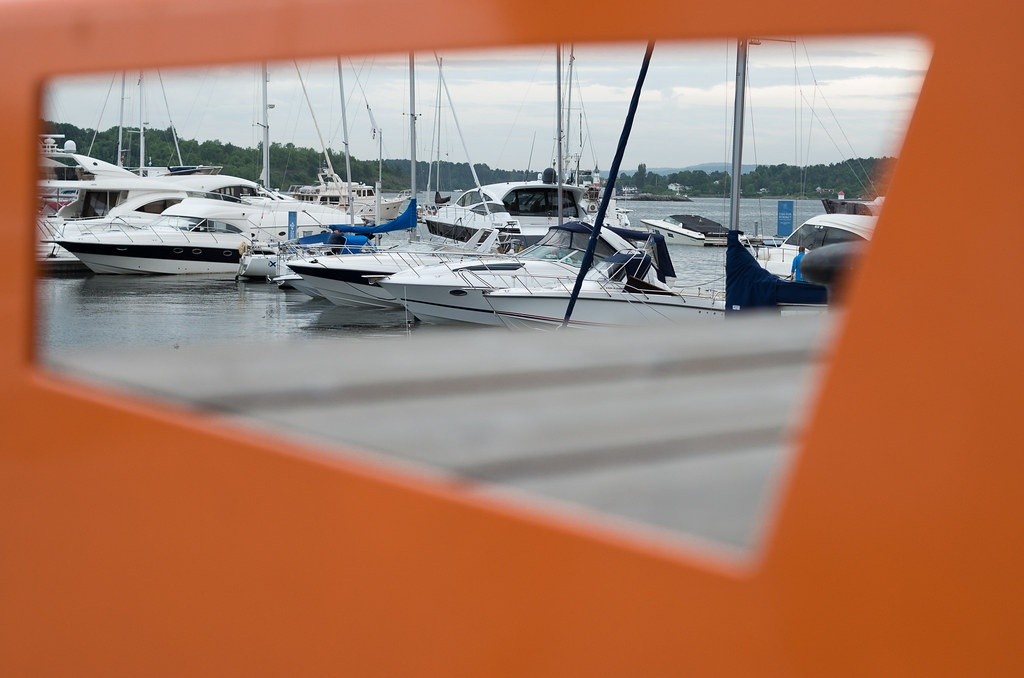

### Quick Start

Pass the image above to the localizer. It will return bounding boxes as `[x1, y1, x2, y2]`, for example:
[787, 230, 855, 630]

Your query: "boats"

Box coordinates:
[640, 213, 784, 247]
[378, 35, 886, 330]
[39, 43, 646, 310]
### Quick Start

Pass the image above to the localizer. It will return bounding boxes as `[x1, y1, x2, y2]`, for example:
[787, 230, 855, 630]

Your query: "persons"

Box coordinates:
[327, 228, 345, 255]
[791, 247, 808, 282]
